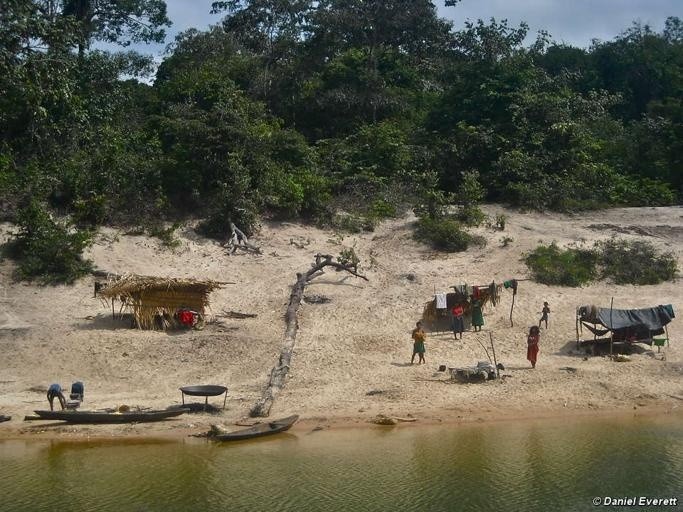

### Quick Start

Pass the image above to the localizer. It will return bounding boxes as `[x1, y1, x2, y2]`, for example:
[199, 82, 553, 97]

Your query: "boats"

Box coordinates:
[213, 415, 297, 440]
[33, 404, 188, 423]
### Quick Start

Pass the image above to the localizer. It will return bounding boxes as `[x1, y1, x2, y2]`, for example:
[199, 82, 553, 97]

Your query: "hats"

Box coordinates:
[530, 326, 539, 336]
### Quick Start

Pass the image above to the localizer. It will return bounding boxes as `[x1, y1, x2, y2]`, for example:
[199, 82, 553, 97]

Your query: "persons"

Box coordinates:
[46, 383, 67, 411]
[409, 321, 425, 363]
[525, 325, 540, 368]
[450, 299, 464, 340]
[536, 301, 550, 329]
[470, 296, 484, 332]
